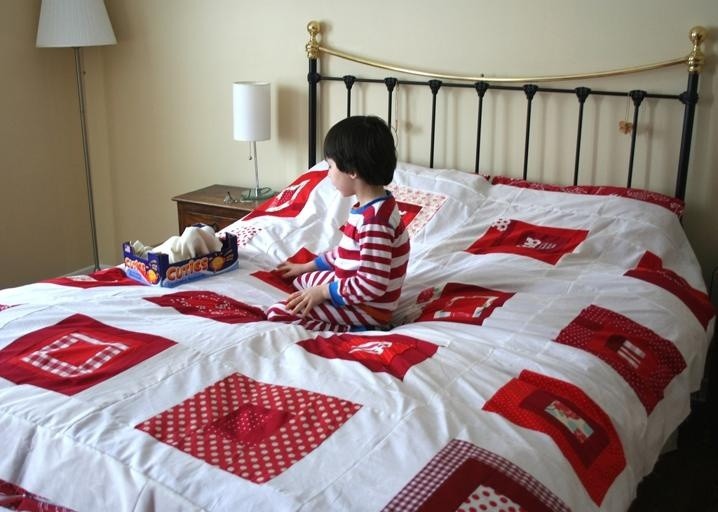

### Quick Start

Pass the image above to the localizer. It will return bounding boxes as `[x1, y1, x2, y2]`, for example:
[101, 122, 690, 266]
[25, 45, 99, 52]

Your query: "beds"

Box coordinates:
[1, 18, 712, 512]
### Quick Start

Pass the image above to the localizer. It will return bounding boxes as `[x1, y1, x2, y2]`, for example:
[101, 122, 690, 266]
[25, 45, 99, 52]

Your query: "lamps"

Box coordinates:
[33, 1, 121, 279]
[228, 78, 279, 205]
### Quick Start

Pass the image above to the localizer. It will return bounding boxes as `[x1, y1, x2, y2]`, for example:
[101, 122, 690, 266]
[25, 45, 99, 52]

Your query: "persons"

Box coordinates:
[265, 115, 410, 332]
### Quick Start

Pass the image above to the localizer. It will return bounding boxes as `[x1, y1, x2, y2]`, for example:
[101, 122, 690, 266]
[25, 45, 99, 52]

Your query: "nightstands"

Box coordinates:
[171, 184, 279, 235]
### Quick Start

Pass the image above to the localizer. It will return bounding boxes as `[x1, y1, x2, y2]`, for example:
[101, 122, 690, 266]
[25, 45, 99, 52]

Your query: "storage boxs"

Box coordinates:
[121, 221, 239, 288]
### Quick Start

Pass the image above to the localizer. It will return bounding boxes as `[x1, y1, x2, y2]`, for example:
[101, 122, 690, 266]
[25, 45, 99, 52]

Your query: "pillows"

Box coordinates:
[489, 175, 685, 223]
